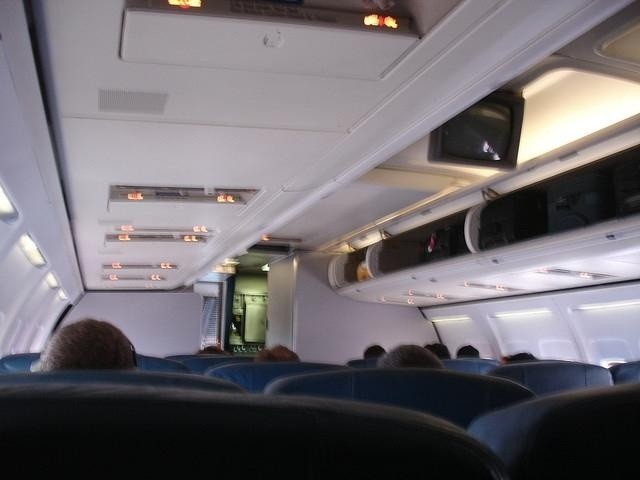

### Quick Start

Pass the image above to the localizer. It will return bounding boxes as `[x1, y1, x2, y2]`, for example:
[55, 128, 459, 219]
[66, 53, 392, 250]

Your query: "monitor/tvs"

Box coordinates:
[428, 92, 527, 170]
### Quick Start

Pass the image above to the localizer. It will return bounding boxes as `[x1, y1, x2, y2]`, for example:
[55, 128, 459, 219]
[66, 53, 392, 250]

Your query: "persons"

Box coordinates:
[456, 345, 479, 358]
[31, 318, 136, 371]
[376, 345, 444, 369]
[423, 343, 450, 360]
[501, 353, 537, 364]
[255, 345, 300, 362]
[364, 344, 386, 359]
[195, 346, 231, 356]
